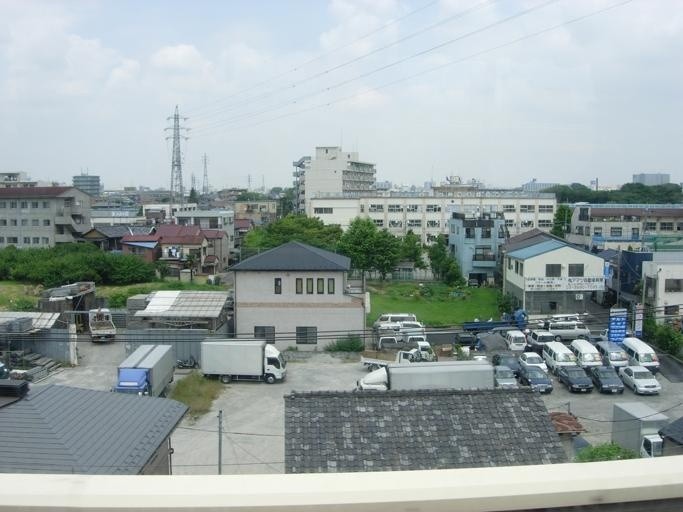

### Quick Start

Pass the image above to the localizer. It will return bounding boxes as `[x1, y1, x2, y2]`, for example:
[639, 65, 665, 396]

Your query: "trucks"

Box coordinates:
[464, 310, 528, 332]
[200, 338, 289, 383]
[360, 351, 417, 371]
[355, 361, 494, 392]
[88, 306, 117, 342]
[109, 345, 175, 398]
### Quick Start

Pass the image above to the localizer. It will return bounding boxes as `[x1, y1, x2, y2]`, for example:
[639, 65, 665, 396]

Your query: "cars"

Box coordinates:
[619, 366, 662, 396]
[494, 366, 518, 392]
[519, 366, 553, 393]
[494, 352, 522, 375]
[589, 365, 624, 393]
[558, 366, 593, 393]
[519, 350, 547, 374]
[468, 278, 479, 289]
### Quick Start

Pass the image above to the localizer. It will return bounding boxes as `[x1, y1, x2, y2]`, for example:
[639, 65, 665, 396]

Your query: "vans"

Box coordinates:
[373, 312, 436, 362]
[504, 312, 660, 374]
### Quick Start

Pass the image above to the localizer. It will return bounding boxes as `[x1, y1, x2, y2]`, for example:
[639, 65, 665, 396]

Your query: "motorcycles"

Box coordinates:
[176, 354, 199, 369]
